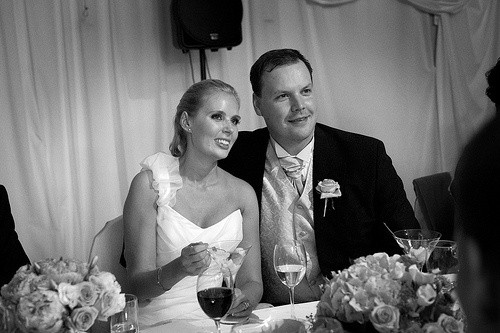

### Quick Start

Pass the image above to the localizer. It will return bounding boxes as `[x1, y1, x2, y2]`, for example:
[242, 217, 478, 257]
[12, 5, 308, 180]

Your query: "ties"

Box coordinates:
[282, 156, 305, 196]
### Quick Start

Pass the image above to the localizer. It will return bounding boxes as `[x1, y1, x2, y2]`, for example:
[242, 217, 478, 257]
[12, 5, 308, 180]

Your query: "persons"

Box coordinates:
[450, 57, 500, 333]
[119, 49, 421, 307]
[123, 79, 263, 333]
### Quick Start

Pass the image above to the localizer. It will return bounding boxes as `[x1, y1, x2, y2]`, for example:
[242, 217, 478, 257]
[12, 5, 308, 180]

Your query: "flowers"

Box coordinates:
[316, 178, 342, 218]
[0, 256, 126, 333]
[305, 252, 467, 333]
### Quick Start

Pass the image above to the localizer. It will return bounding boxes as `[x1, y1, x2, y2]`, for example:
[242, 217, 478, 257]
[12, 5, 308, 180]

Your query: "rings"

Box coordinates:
[242, 301, 248, 309]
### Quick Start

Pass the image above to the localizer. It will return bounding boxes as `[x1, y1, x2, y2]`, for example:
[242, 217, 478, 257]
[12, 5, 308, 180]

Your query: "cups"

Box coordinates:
[393, 228, 441, 271]
[425, 240, 458, 284]
[111, 293, 139, 333]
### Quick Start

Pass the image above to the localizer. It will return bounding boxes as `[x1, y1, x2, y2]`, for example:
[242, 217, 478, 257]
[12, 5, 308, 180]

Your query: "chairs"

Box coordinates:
[413, 172, 453, 267]
[88, 215, 124, 296]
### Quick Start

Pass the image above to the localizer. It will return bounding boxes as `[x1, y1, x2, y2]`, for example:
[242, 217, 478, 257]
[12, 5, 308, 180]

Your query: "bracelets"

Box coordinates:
[156, 268, 171, 291]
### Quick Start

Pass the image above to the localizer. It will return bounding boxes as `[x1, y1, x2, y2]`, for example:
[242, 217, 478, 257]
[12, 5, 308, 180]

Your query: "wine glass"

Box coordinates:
[273, 242, 310, 330]
[197, 266, 234, 333]
[208, 240, 254, 324]
